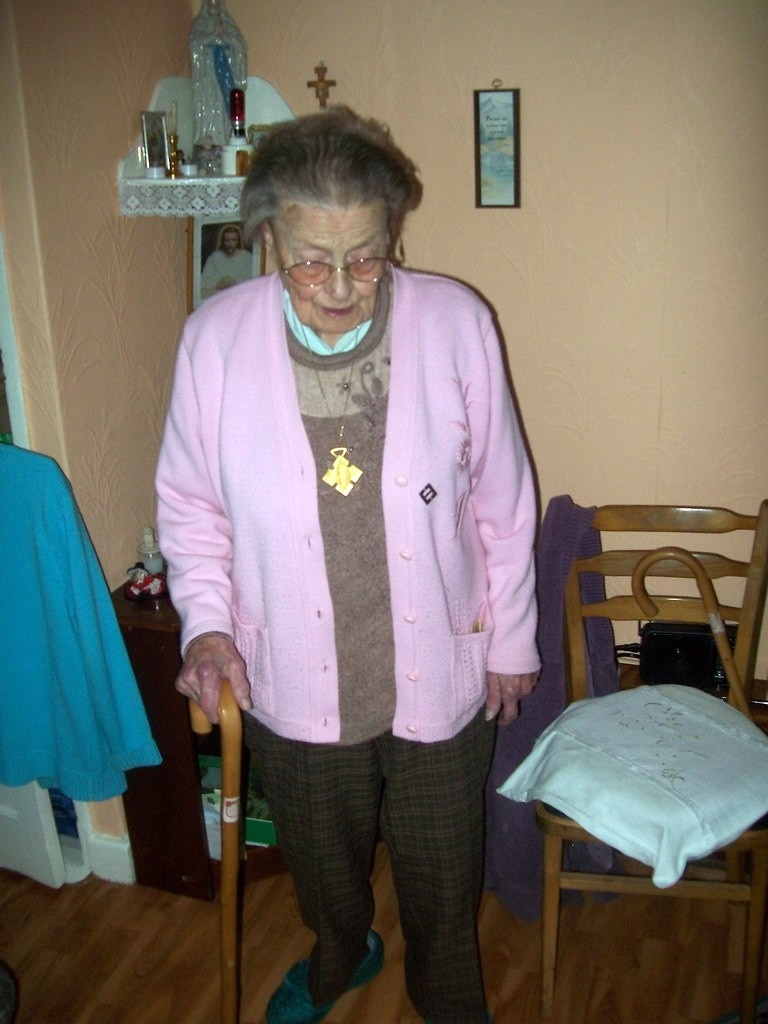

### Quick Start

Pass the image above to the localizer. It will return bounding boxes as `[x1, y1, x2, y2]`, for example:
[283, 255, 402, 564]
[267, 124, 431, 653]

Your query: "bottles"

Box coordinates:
[136, 526, 165, 575]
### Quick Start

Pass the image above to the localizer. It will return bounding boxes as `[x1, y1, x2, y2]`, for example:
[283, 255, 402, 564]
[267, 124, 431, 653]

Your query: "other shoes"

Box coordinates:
[266, 928, 385, 1024]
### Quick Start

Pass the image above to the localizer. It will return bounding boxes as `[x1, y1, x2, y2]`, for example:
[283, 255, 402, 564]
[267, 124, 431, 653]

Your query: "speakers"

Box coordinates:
[638, 623, 738, 689]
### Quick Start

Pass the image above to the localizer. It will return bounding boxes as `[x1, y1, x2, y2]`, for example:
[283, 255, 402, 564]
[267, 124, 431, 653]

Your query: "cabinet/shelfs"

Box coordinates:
[113, 573, 276, 902]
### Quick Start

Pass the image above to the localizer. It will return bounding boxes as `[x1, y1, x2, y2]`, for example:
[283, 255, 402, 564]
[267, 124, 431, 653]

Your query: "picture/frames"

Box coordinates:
[472, 88, 522, 210]
[185, 214, 268, 320]
[140, 110, 171, 176]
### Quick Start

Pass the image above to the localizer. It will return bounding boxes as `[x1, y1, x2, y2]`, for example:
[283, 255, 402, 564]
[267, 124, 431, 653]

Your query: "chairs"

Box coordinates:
[536, 495, 768, 1024]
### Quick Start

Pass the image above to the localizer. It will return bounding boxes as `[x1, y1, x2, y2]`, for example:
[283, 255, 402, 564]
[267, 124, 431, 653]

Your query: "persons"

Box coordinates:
[154, 106, 543, 1024]
[188, 0, 248, 146]
[201, 224, 254, 288]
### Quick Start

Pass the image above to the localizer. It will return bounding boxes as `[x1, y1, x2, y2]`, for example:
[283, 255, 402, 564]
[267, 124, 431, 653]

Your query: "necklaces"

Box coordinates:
[302, 325, 364, 497]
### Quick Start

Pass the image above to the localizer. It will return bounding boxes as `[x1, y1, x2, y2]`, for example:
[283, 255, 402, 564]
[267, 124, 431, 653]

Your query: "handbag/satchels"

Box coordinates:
[637, 624, 737, 691]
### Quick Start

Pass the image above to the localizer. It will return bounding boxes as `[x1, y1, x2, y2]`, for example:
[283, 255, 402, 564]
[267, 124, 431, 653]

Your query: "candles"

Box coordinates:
[170, 100, 178, 135]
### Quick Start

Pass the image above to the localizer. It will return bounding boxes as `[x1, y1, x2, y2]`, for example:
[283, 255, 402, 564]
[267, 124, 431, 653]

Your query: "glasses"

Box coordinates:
[268, 222, 393, 287]
